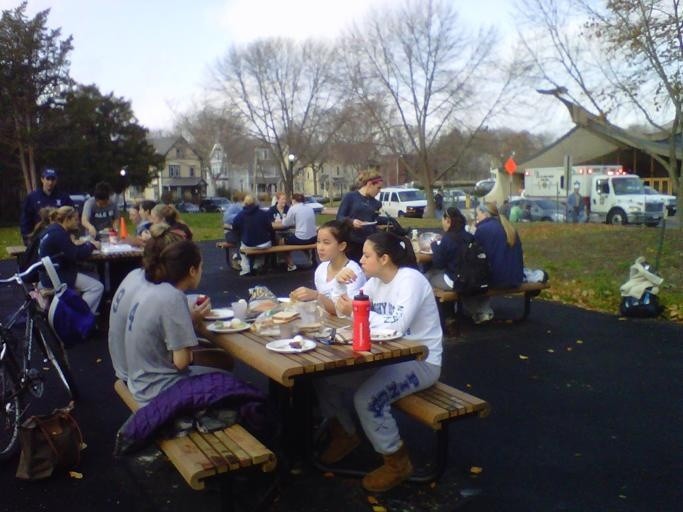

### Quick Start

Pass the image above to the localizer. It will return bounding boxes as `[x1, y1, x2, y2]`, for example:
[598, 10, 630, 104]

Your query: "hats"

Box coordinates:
[41, 169, 57, 178]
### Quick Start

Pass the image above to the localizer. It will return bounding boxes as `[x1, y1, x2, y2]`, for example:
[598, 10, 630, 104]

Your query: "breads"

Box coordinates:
[300, 322, 323, 332]
[272, 311, 299, 318]
[273, 317, 301, 324]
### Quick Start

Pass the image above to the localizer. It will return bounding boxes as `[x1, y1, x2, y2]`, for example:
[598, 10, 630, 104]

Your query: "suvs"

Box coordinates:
[199, 197, 233, 213]
[628, 185, 677, 217]
[304, 195, 324, 214]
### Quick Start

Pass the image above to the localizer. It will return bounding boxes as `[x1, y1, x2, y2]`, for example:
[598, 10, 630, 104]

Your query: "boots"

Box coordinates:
[361, 442, 415, 492]
[321, 415, 361, 466]
[444, 317, 456, 337]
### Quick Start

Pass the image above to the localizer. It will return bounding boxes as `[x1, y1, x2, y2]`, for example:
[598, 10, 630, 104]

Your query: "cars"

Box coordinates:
[498, 198, 567, 223]
[176, 202, 200, 213]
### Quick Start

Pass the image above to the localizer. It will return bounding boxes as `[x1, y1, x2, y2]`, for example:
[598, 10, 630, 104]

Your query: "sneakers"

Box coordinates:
[287, 263, 297, 272]
[239, 268, 251, 276]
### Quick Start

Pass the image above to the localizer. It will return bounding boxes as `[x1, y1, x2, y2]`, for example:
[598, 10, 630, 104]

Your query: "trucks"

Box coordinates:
[523, 164, 668, 226]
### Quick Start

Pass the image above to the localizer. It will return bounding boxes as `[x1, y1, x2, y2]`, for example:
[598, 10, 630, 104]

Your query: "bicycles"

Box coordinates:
[1, 251, 79, 461]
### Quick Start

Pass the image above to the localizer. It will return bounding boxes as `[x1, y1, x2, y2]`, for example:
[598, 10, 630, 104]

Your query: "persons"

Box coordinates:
[17, 206, 56, 283]
[37, 206, 105, 316]
[500, 199, 532, 223]
[108, 223, 210, 385]
[232, 195, 273, 276]
[269, 192, 289, 245]
[283, 193, 317, 272]
[133, 203, 141, 225]
[81, 182, 120, 240]
[317, 232, 443, 491]
[136, 200, 156, 232]
[222, 197, 243, 270]
[125, 240, 234, 407]
[566, 184, 585, 223]
[336, 170, 384, 261]
[434, 189, 443, 219]
[463, 201, 524, 325]
[151, 203, 192, 239]
[292, 219, 366, 319]
[428, 207, 474, 325]
[20, 168, 74, 246]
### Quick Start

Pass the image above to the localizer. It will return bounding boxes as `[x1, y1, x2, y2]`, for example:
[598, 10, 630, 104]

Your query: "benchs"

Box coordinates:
[114, 374, 277, 492]
[214, 240, 240, 269]
[4, 242, 27, 279]
[432, 279, 551, 323]
[239, 243, 317, 277]
[392, 383, 492, 484]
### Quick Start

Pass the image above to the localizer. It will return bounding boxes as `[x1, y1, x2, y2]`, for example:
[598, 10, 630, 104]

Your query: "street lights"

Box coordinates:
[288, 154, 295, 199]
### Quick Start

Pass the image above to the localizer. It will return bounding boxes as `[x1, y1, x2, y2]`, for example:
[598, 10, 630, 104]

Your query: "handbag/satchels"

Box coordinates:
[15, 399, 85, 481]
[41, 255, 106, 365]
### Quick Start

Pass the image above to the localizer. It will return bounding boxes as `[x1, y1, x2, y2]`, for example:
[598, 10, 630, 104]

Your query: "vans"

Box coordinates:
[375, 188, 427, 218]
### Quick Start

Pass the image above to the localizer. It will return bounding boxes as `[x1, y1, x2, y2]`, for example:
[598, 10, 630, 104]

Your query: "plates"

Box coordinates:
[203, 309, 235, 320]
[266, 338, 317, 354]
[206, 321, 252, 334]
[369, 329, 403, 342]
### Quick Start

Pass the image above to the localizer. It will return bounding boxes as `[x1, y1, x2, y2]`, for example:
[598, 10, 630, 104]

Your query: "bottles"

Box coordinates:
[352, 291, 372, 351]
[109, 227, 117, 245]
[410, 229, 420, 253]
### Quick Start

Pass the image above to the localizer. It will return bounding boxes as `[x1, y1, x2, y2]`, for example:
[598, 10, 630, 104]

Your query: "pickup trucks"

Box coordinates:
[442, 189, 472, 203]
